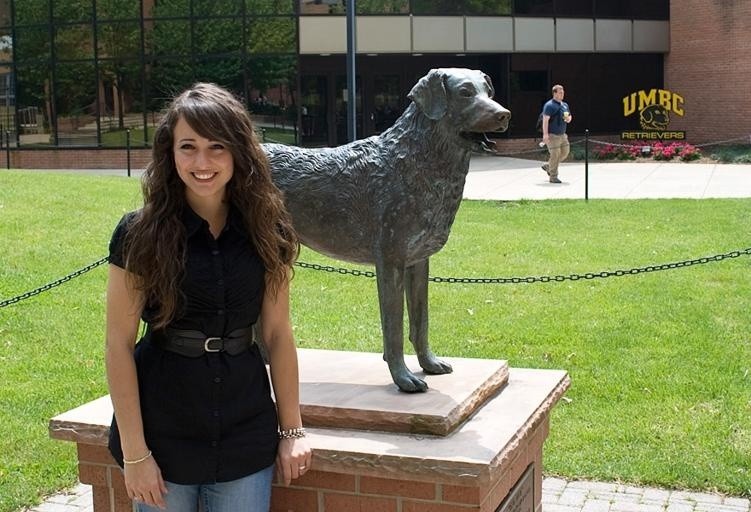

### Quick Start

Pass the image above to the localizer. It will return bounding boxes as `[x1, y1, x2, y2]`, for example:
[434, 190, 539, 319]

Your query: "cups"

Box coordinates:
[563, 111, 569, 121]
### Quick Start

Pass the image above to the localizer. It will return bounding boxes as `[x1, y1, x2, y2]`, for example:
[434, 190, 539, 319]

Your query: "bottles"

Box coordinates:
[539, 142, 545, 147]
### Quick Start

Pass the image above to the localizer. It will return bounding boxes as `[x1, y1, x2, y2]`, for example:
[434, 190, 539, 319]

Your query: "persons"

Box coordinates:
[103, 83, 311, 512]
[542, 84, 573, 183]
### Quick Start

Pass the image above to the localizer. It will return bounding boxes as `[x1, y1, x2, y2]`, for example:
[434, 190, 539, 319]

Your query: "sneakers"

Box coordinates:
[542, 164, 561, 183]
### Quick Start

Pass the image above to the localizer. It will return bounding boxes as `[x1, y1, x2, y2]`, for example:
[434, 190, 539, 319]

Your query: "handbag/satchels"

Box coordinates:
[536, 110, 553, 133]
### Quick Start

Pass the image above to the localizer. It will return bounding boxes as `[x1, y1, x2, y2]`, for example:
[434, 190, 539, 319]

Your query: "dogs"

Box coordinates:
[258, 67, 512, 393]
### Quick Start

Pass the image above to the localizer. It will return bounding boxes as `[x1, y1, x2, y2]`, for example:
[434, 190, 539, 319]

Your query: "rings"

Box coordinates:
[299, 466, 305, 470]
[136, 496, 143, 501]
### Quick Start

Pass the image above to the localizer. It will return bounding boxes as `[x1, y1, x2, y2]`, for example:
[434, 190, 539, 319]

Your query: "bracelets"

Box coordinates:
[123, 451, 151, 465]
[280, 427, 305, 439]
